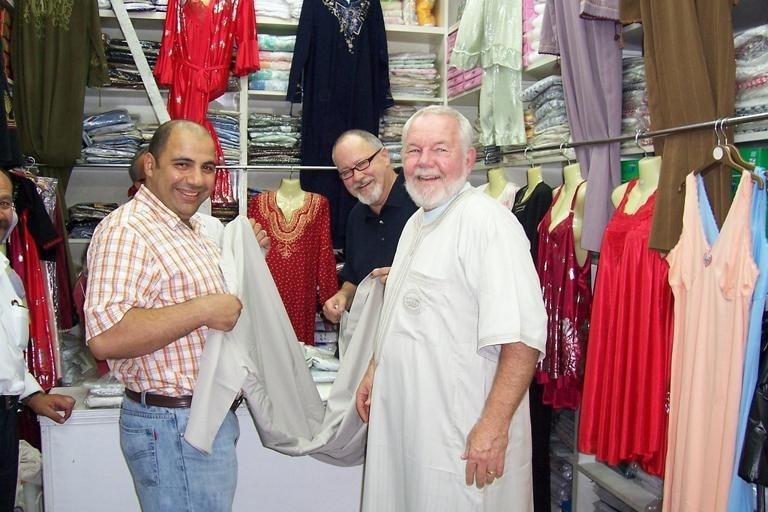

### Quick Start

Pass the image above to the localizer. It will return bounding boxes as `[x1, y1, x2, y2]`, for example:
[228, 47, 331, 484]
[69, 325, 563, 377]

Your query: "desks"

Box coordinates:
[37, 384, 367, 512]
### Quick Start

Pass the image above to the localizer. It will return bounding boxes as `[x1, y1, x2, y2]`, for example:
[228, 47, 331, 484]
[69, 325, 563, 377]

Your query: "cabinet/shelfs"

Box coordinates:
[69, 0, 653, 245]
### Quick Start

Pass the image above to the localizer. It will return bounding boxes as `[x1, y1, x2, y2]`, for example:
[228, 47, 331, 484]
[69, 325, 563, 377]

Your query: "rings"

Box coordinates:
[487, 471, 497, 475]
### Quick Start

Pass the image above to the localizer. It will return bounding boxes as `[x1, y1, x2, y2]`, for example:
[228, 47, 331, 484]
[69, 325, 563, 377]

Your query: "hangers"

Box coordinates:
[676, 116, 768, 190]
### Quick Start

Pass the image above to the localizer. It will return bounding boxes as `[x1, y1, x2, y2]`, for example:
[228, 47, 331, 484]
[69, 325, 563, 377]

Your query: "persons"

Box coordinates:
[130, 146, 150, 190]
[578, 156, 674, 478]
[84, 120, 271, 512]
[478, 163, 594, 408]
[355, 105, 548, 512]
[249, 177, 340, 345]
[323, 129, 420, 324]
[0, 170, 76, 512]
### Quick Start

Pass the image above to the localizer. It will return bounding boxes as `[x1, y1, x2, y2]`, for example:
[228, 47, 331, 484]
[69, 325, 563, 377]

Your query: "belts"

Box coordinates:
[125, 387, 243, 413]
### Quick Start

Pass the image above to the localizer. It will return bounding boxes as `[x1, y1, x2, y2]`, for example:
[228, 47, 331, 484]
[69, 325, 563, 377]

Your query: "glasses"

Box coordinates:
[338, 147, 383, 180]
